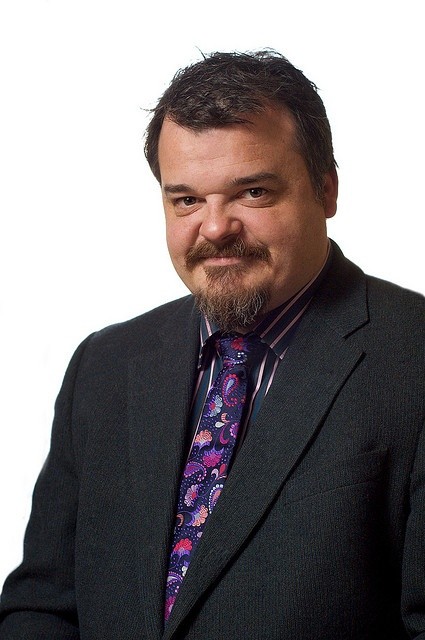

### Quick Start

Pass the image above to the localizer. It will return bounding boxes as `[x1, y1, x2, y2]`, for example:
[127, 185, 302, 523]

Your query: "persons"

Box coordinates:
[0, 45, 425, 639]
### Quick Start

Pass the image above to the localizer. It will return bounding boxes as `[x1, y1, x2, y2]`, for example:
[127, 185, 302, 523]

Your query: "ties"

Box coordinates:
[163, 328, 263, 626]
